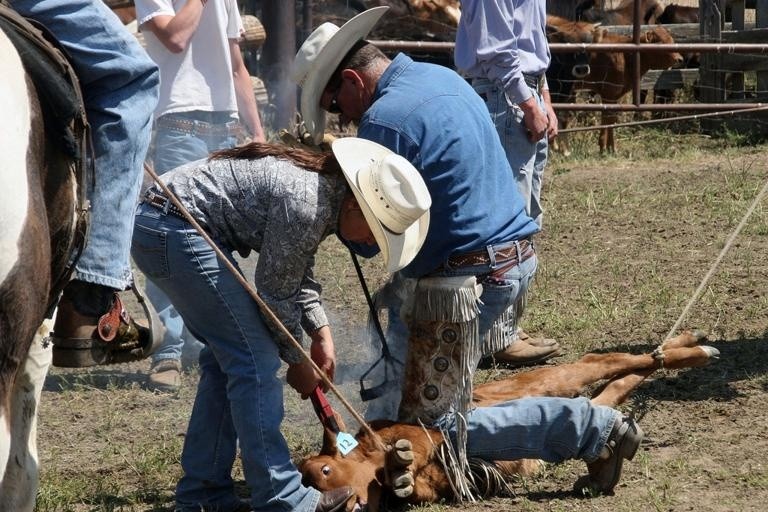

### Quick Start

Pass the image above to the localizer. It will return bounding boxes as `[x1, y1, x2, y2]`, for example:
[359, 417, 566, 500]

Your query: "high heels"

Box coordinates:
[573, 410, 644, 497]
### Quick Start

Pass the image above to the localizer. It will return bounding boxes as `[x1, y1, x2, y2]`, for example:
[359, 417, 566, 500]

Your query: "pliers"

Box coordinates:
[309, 385, 339, 436]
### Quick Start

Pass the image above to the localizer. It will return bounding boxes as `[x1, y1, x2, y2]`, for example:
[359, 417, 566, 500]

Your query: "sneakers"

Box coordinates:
[52, 290, 152, 369]
[147, 358, 182, 397]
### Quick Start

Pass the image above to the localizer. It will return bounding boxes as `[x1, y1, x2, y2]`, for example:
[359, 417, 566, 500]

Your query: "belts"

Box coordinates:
[432, 237, 533, 276]
[156, 117, 244, 139]
[468, 73, 540, 91]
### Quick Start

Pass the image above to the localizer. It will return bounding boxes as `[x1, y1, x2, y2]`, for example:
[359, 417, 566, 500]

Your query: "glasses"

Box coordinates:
[328, 76, 341, 116]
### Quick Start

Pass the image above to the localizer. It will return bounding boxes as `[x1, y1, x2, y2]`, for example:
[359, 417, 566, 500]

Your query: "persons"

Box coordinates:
[125, 130, 434, 512]
[130, 0, 268, 396]
[10, 0, 152, 368]
[451, 0, 564, 371]
[290, 6, 644, 499]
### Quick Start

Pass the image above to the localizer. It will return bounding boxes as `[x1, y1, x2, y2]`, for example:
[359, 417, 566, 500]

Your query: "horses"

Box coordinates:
[0, 3, 80, 512]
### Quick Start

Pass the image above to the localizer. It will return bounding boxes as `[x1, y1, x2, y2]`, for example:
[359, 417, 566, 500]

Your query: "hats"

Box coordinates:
[289, 3, 391, 146]
[330, 134, 434, 274]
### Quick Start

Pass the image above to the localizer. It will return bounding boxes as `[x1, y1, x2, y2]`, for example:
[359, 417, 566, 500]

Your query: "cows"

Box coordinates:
[313, 0, 701, 159]
[297, 329, 721, 512]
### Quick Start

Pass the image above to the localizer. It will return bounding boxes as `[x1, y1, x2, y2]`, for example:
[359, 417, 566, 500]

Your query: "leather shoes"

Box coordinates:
[480, 330, 560, 369]
[317, 486, 356, 512]
[183, 495, 254, 512]
